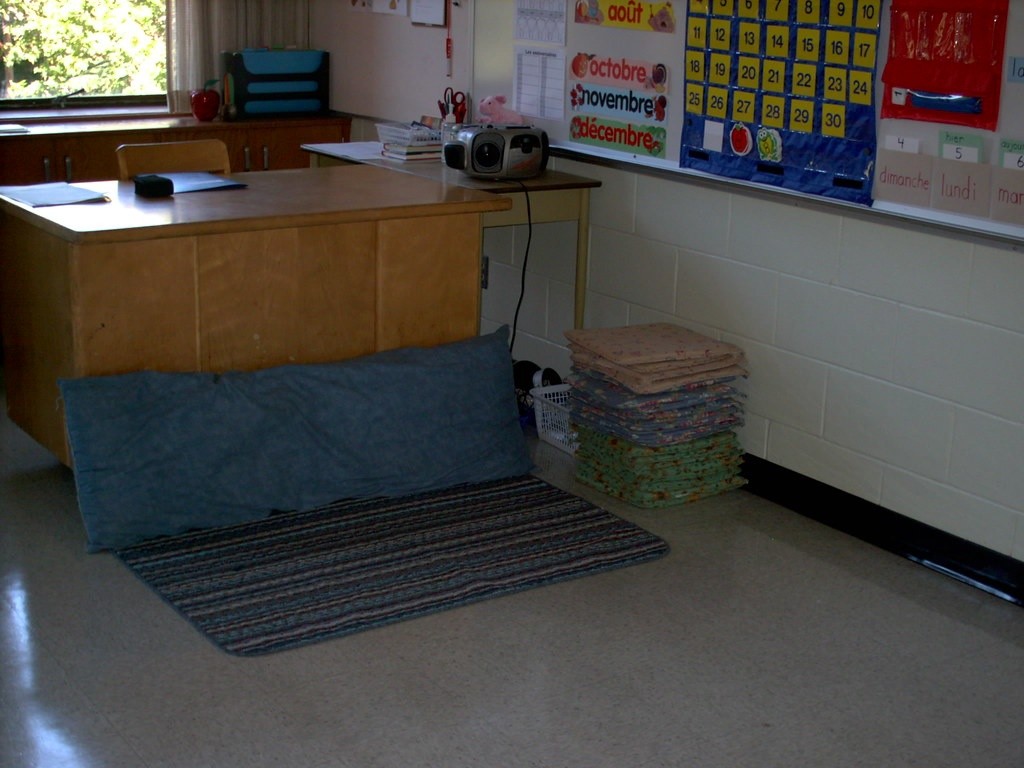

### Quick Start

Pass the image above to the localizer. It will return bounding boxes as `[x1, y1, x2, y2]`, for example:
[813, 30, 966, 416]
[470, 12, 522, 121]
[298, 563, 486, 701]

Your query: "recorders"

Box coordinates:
[443, 121, 550, 181]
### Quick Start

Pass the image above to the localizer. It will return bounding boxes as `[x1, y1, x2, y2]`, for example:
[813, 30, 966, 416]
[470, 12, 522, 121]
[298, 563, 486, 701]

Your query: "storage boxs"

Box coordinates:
[529, 382, 581, 456]
[219, 48, 329, 117]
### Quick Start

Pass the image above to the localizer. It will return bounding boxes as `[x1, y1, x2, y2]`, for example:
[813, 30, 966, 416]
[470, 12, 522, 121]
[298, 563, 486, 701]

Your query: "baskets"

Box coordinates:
[529, 384, 580, 456]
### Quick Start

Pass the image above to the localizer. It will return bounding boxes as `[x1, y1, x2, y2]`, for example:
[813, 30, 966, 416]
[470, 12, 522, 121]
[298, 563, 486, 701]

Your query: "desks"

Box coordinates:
[299, 141, 603, 330]
[0, 163, 514, 473]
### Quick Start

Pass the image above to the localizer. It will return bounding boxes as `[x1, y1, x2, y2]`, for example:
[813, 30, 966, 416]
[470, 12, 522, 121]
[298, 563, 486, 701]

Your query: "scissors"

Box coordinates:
[444, 88, 464, 115]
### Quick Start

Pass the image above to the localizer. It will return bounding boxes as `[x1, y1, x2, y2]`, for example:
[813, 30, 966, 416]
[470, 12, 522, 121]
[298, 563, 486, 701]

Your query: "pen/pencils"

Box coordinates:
[437, 87, 468, 124]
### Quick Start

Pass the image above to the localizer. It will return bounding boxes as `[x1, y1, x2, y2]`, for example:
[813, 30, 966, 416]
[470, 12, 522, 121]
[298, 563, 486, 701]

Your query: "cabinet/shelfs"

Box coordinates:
[0, 109, 353, 187]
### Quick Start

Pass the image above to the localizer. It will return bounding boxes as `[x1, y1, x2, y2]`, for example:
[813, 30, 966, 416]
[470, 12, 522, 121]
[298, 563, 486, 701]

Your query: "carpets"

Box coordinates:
[107, 471, 672, 656]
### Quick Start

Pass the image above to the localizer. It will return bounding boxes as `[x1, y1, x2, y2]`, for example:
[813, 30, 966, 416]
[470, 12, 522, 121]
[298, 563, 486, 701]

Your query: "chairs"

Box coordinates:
[114, 139, 234, 181]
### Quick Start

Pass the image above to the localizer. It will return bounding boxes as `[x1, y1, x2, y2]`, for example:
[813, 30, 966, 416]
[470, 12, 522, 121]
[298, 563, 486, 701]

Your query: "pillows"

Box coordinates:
[55, 321, 539, 555]
